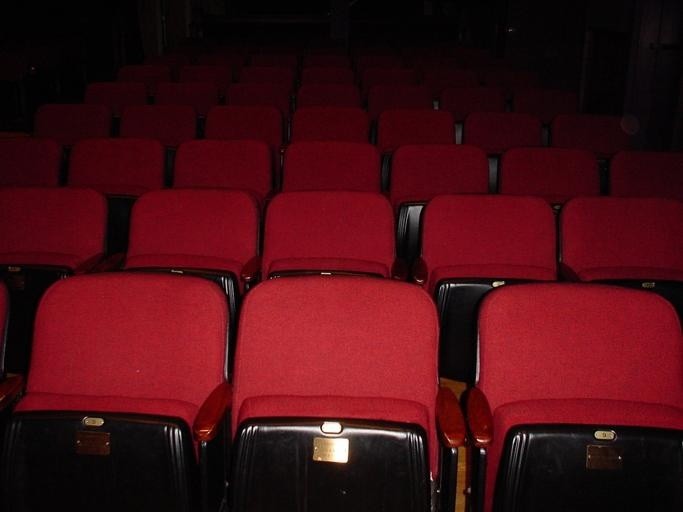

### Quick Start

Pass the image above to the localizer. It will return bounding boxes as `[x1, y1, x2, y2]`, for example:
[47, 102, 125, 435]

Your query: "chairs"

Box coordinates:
[378, 107, 455, 143]
[121, 100, 197, 182]
[1, 271, 230, 512]
[201, 106, 279, 141]
[559, 196, 586, 282]
[122, 188, 259, 287]
[192, 276, 466, 510]
[171, 140, 272, 199]
[389, 142, 489, 278]
[411, 192, 558, 284]
[0, 137, 59, 185]
[466, 284, 683, 512]
[1, 186, 107, 274]
[460, 112, 546, 187]
[497, 147, 600, 203]
[607, 151, 682, 198]
[289, 104, 369, 141]
[260, 191, 396, 278]
[66, 136, 164, 262]
[85, 53, 586, 114]
[279, 141, 381, 192]
[555, 114, 643, 150]
[40, 100, 114, 135]
